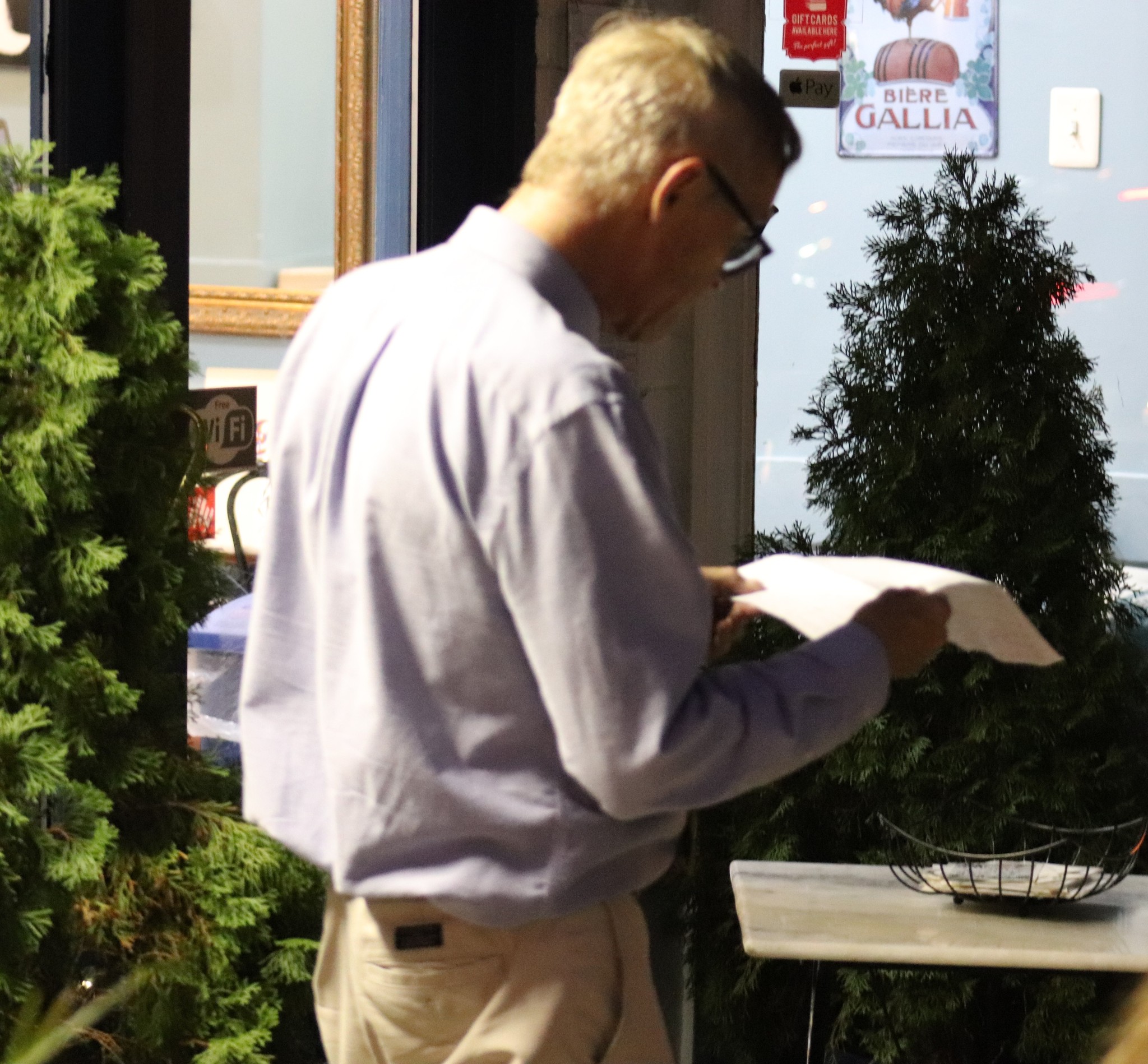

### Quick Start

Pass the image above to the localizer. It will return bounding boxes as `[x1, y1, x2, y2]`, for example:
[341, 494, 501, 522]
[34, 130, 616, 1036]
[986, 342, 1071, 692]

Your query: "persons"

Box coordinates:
[236, 11, 951, 1064]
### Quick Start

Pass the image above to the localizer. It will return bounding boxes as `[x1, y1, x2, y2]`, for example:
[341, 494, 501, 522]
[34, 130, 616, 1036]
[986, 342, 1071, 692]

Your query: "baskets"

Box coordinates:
[877, 808, 1148, 920]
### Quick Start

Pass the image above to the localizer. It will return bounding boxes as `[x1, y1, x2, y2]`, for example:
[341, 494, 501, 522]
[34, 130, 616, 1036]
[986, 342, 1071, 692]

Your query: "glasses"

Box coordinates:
[700, 159, 774, 278]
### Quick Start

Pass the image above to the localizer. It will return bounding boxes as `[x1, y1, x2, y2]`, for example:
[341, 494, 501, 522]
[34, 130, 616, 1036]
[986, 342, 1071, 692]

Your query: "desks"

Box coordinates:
[728, 860, 1148, 1064]
